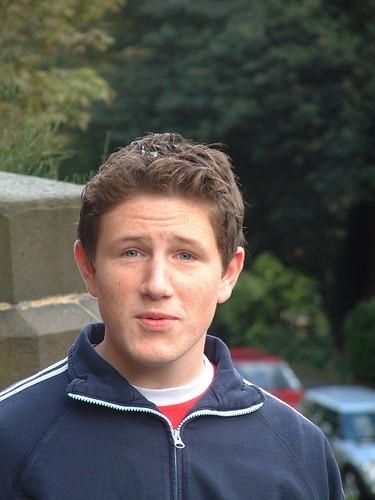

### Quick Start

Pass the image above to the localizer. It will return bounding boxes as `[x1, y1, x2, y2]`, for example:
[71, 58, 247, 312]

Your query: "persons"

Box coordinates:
[0, 130, 347, 500]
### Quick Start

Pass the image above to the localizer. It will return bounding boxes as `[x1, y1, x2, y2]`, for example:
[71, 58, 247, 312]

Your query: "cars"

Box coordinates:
[304, 383, 375, 500]
[228, 345, 303, 414]
